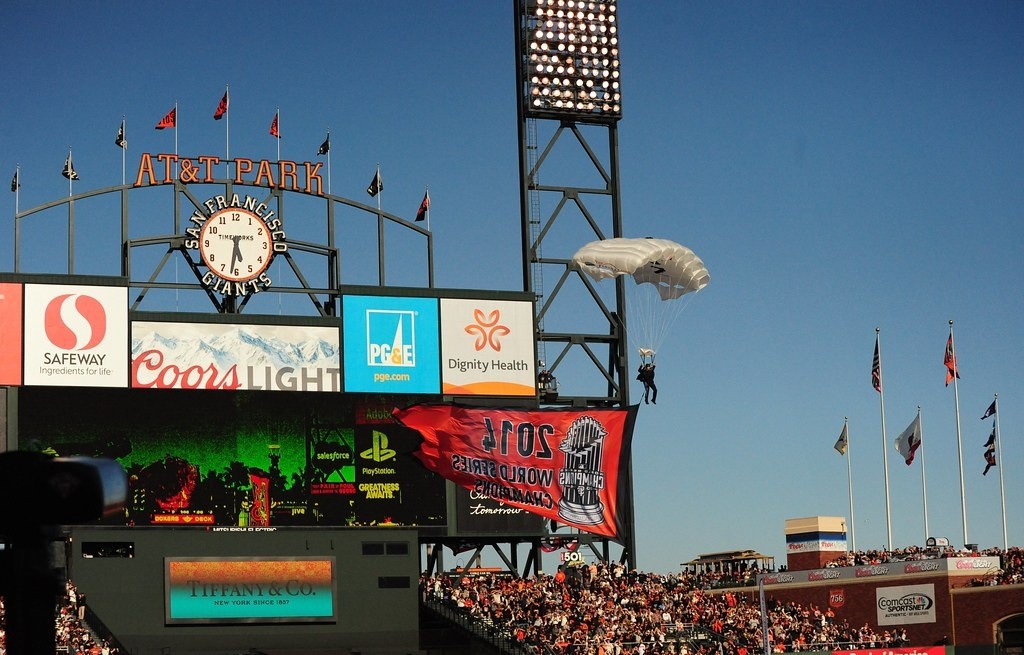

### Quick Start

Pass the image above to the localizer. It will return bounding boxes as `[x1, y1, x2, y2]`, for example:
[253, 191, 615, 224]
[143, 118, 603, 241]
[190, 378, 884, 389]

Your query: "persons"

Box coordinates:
[416, 551, 911, 655]
[54, 576, 121, 655]
[538, 370, 557, 389]
[825, 545, 1024, 588]
[636, 361, 658, 405]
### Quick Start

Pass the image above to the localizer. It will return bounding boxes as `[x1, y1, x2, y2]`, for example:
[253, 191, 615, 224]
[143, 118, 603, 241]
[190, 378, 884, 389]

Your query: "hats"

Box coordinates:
[646, 364, 650, 367]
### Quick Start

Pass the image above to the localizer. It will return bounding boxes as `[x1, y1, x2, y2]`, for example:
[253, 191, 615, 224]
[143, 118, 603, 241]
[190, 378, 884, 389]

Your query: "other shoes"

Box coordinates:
[651, 399, 656, 404]
[645, 399, 649, 404]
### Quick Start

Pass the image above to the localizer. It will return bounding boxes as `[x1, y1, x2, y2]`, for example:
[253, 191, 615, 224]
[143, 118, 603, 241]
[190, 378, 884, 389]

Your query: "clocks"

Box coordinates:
[180, 192, 289, 303]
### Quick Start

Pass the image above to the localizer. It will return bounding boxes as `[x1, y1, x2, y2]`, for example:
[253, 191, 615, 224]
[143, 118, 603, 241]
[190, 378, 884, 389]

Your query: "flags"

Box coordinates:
[871, 333, 882, 392]
[269, 113, 282, 138]
[894, 413, 922, 467]
[213, 90, 230, 120]
[317, 137, 330, 156]
[155, 107, 176, 130]
[834, 423, 848, 456]
[11, 171, 20, 192]
[62, 153, 80, 180]
[414, 191, 430, 222]
[980, 399, 1002, 476]
[367, 171, 383, 197]
[115, 122, 128, 151]
[943, 332, 961, 388]
